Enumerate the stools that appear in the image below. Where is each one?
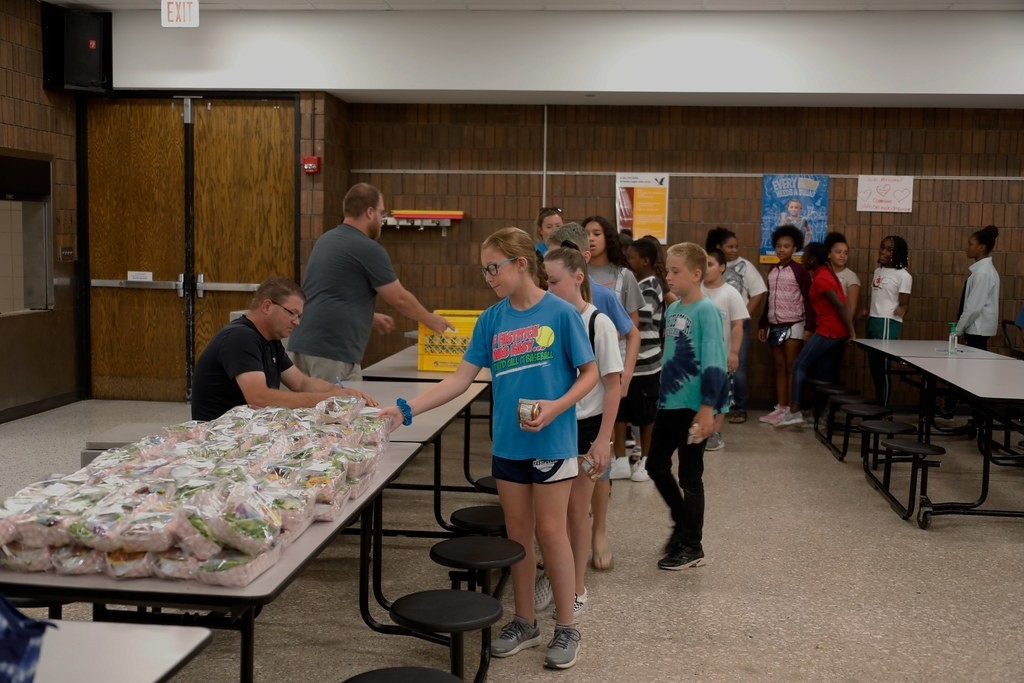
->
[807,378,946,520]
[475,476,498,495]
[429,536,526,595]
[451,506,508,539]
[342,667,464,683]
[389,589,503,679]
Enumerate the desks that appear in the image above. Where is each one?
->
[851,340,1024,530]
[1,342,492,683]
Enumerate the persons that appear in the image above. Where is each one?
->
[780,199,813,248]
[286,183,455,384]
[699,225,861,450]
[533,207,728,622]
[376,228,599,670]
[855,236,912,408]
[192,277,378,422]
[935,224,999,422]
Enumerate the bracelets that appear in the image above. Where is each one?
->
[397,398,412,426]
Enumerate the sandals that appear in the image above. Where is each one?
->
[729,411,747,424]
[714,412,731,419]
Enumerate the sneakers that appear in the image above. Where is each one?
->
[663,522,680,555]
[630,456,650,481]
[553,586,587,619]
[705,432,724,450]
[533,576,553,610]
[609,456,631,479]
[760,404,791,424]
[934,413,955,423]
[773,411,804,429]
[800,416,826,429]
[658,544,706,570]
[490,618,542,657]
[544,623,581,668]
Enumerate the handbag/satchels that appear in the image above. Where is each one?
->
[767,327,787,347]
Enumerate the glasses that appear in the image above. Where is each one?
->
[480,256,518,277]
[271,300,303,322]
[540,207,562,216]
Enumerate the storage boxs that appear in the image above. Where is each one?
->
[418,309,484,371]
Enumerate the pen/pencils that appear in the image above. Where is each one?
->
[336,377,344,389]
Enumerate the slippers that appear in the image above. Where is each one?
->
[592,545,614,573]
[537,559,544,569]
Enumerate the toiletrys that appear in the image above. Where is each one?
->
[947,322,958,354]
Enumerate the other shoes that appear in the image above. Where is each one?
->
[629,446,642,465]
[625,425,636,448]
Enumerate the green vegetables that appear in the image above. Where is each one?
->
[0,401,388,572]
